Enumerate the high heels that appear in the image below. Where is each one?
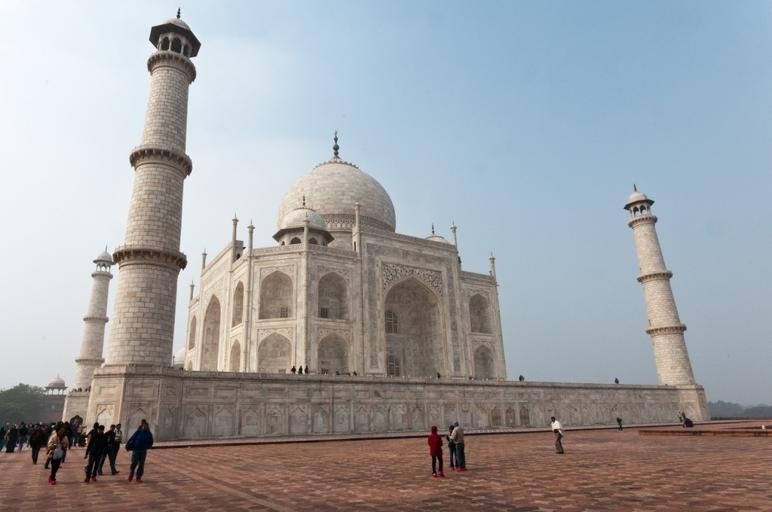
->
[49,475,56,485]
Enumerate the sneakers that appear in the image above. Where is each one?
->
[128,476,133,481]
[438,470,444,477]
[85,477,90,481]
[136,478,144,482]
[92,476,97,481]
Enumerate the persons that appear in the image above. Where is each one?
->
[299,366,302,374]
[519,376,524,381]
[126,420,153,483]
[428,426,444,477]
[449,422,465,472]
[679,412,693,427]
[305,366,308,374]
[447,425,457,468]
[291,366,296,373]
[550,417,564,454]
[617,418,623,431]
[615,378,619,384]
[0,421,123,486]
[437,373,440,379]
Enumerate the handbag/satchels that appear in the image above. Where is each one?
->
[126,430,140,450]
[53,444,64,460]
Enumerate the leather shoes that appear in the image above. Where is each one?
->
[99,472,102,475]
[112,471,119,475]
